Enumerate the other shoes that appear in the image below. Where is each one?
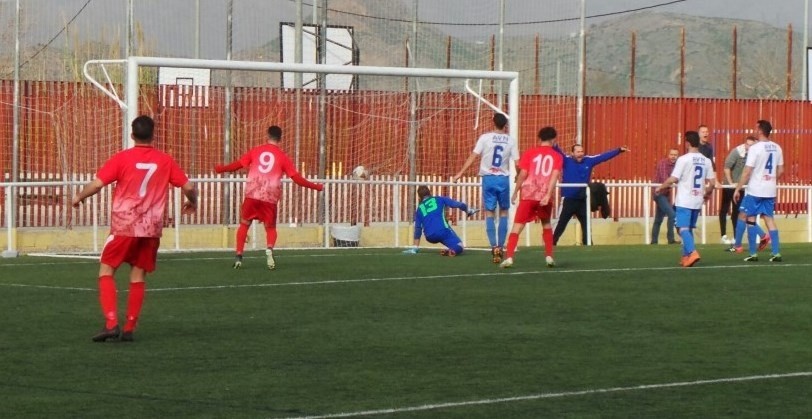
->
[668,240,681,244]
[440,249,456,257]
[492,247,502,263]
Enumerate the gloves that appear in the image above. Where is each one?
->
[403,249,418,255]
[466,208,479,216]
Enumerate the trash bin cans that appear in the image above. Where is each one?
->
[333,224,361,247]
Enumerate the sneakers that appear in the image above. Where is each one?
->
[122,331,132,341]
[545,256,555,267]
[266,247,275,270]
[725,246,742,253]
[757,233,771,251]
[93,323,120,342]
[721,235,736,246]
[745,254,758,262]
[769,254,781,262]
[500,258,513,268]
[680,251,701,267]
[234,256,241,269]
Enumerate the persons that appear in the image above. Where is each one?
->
[655,131,715,268]
[499,126,563,269]
[719,119,785,263]
[697,125,714,163]
[71,115,199,343]
[650,148,682,245]
[553,141,631,246]
[401,187,479,257]
[453,113,520,262]
[213,125,323,270]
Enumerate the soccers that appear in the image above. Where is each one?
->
[353,166,368,180]
